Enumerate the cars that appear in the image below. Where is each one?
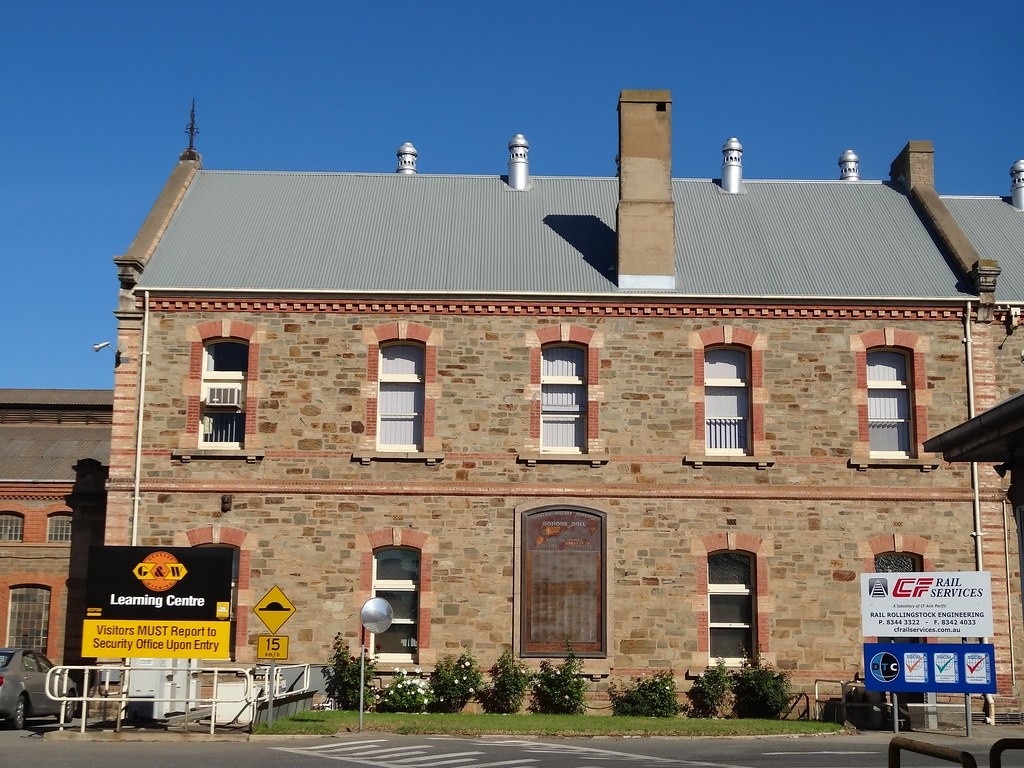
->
[0,648,79,729]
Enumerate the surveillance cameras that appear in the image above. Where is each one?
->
[93,341,110,352]
